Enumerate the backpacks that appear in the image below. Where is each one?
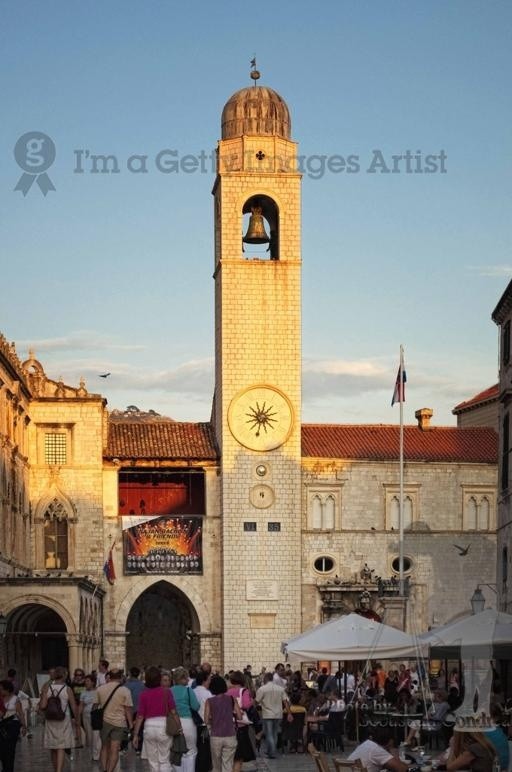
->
[47,686,66,721]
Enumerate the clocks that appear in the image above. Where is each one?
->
[227,383,295,452]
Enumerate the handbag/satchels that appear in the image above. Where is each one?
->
[90,707,104,730]
[166,712,179,737]
[191,709,203,725]
[233,708,254,728]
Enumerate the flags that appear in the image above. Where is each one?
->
[390,355,408,407]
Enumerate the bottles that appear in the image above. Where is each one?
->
[128,550,199,571]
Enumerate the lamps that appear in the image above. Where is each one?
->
[470,583,498,614]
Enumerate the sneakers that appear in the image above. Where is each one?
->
[400,742,411,747]
[410,745,425,752]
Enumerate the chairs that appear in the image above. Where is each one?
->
[252,699,512,772]
